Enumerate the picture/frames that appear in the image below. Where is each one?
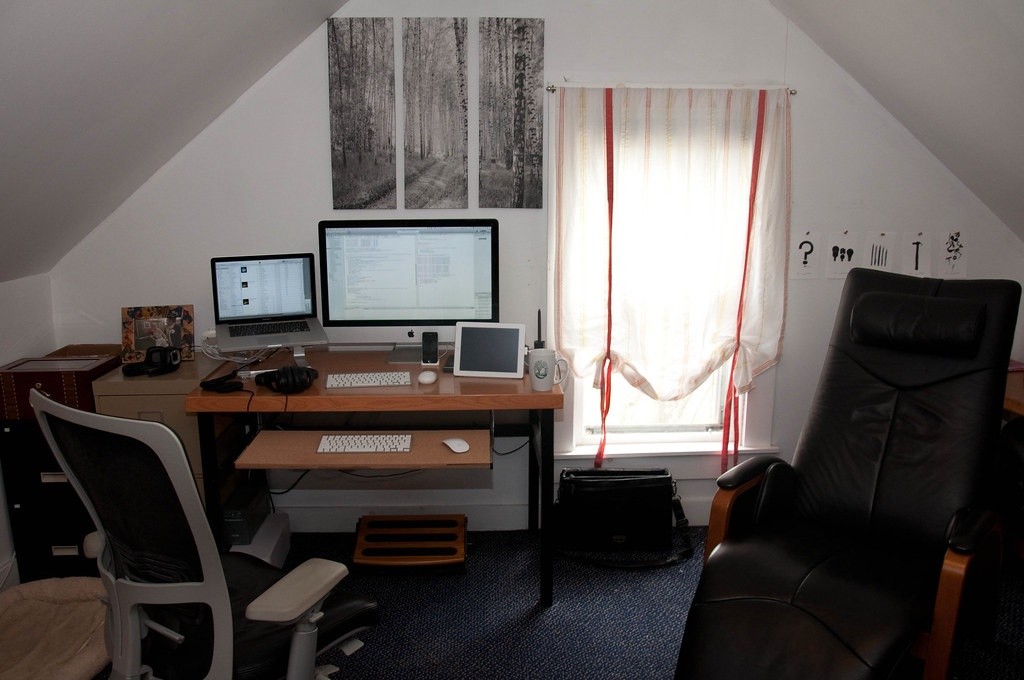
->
[120,305,195,363]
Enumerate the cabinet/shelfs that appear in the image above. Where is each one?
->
[1,415,120,582]
[92,352,234,509]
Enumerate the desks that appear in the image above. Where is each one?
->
[187,345,564,606]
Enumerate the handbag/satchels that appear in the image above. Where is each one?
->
[553,469,707,574]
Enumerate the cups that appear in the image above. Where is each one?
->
[528,349,569,391]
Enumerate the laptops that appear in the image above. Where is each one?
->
[211,252,329,352]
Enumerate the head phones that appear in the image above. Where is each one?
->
[255,366,318,395]
[122,345,181,377]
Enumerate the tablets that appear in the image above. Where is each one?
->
[453,322,526,378]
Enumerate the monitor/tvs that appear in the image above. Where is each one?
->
[318,218,499,363]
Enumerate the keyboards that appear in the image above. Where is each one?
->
[317,435,412,452]
[325,371,411,386]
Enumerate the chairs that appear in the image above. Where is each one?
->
[672,267,1023,680]
[30,389,378,679]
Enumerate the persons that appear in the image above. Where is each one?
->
[150,318,180,348]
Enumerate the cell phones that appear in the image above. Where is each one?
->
[422,332,438,363]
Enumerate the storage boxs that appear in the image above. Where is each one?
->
[0,357,119,420]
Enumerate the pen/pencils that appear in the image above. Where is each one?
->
[236,368,278,376]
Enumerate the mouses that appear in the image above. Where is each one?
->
[442,439,469,453]
[418,371,437,384]
